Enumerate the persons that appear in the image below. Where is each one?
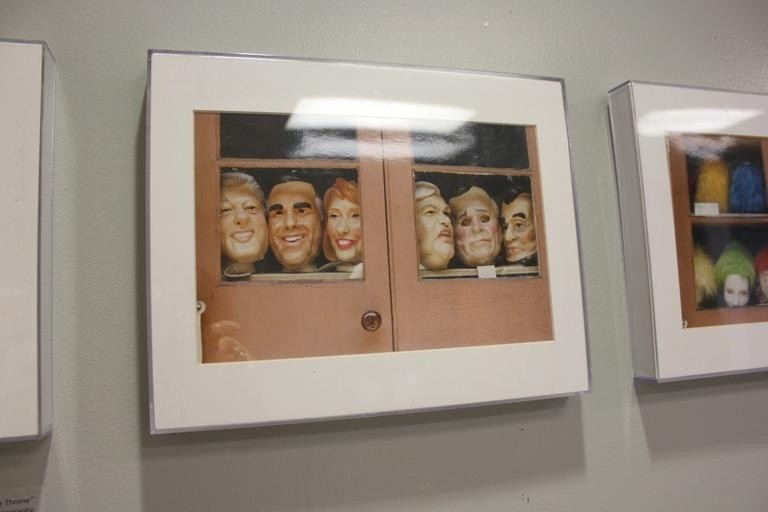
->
[219,170,271,266]
[414,181,455,270]
[448,184,503,268]
[713,239,756,307]
[263,174,323,274]
[208,320,249,362]
[320,177,364,264]
[499,186,538,263]
[752,250,768,304]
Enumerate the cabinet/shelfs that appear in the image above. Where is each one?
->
[192,109,556,367]
[664,130,768,328]
